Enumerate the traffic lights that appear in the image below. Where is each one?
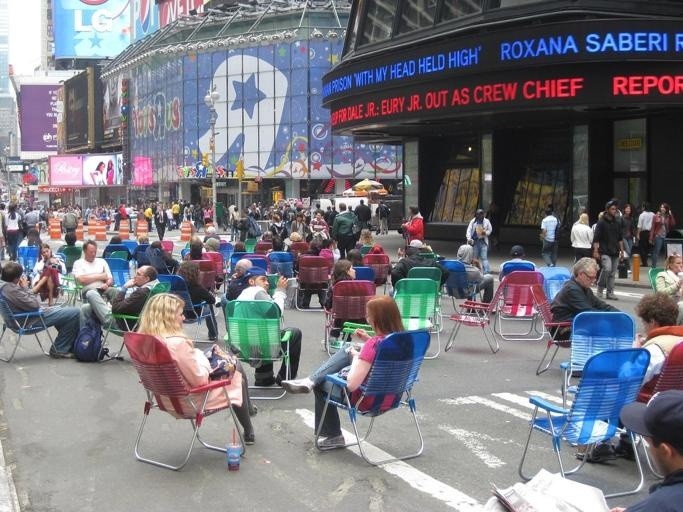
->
[237,160,244,178]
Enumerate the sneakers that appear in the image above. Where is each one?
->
[316,436,346,451]
[606,289,618,300]
[627,270,632,274]
[597,286,605,298]
[50,350,75,358]
[280,377,313,393]
[249,404,258,416]
[244,433,255,444]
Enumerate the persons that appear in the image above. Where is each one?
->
[24,245,68,306]
[15,206,27,214]
[63,208,78,234]
[6,204,22,261]
[324,206,332,219]
[465,209,492,274]
[623,205,635,268]
[232,267,302,386]
[92,162,105,185]
[281,295,414,448]
[71,241,123,332]
[402,204,424,254]
[1,204,5,217]
[324,261,365,340]
[204,227,220,244]
[448,245,496,314]
[357,229,373,247]
[165,200,216,230]
[79,266,158,341]
[241,210,249,241]
[249,198,310,214]
[102,237,132,261]
[205,239,224,288]
[170,261,220,339]
[269,214,286,248]
[540,209,561,268]
[85,204,112,224]
[258,232,273,243]
[74,205,82,219]
[265,238,283,258]
[112,202,154,233]
[37,205,62,224]
[145,246,170,270]
[310,212,328,244]
[332,204,360,259]
[578,292,683,462]
[156,205,167,240]
[134,236,148,258]
[142,241,177,264]
[636,202,655,267]
[230,243,250,255]
[217,203,238,225]
[551,256,624,349]
[376,202,389,234]
[612,390,682,511]
[347,205,355,216]
[354,200,371,230]
[214,259,251,333]
[330,205,339,219]
[230,208,241,241]
[577,206,584,213]
[17,229,43,247]
[393,246,449,297]
[185,238,213,287]
[364,245,392,284]
[499,246,536,314]
[132,292,256,445]
[648,203,676,269]
[657,253,681,321]
[320,239,334,277]
[570,213,593,259]
[589,211,604,235]
[345,249,365,269]
[107,160,114,184]
[315,203,324,217]
[0,262,79,360]
[593,199,626,301]
[291,214,306,241]
[25,209,38,233]
[296,240,329,309]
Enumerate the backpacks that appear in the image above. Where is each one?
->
[246,217,262,237]
[73,312,107,361]
[555,217,564,241]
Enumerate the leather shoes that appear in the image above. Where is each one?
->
[575,443,617,463]
[466,309,478,313]
[547,264,555,267]
[479,309,496,314]
[640,264,649,267]
[612,443,636,461]
[254,376,276,387]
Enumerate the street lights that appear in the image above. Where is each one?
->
[4,146,11,203]
[205,80,220,232]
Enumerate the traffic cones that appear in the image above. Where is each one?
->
[51,222,61,239]
[76,224,84,240]
[95,221,107,241]
[119,220,129,239]
[181,223,192,241]
[88,221,95,235]
[49,219,55,234]
[136,221,148,240]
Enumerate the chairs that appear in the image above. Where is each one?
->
[408,267,444,333]
[0,295,63,363]
[324,280,376,357]
[241,254,267,270]
[15,245,43,279]
[440,260,489,316]
[359,245,372,254]
[269,252,294,286]
[159,240,173,257]
[340,322,377,351]
[107,248,130,263]
[132,244,149,254]
[392,277,440,361]
[499,261,537,277]
[195,249,228,295]
[315,330,430,467]
[125,330,246,472]
[494,270,546,344]
[264,275,283,302]
[184,258,218,318]
[180,248,191,257]
[103,257,131,291]
[254,241,277,253]
[111,242,138,256]
[417,253,437,261]
[348,267,374,282]
[218,241,234,269]
[99,279,171,363]
[559,311,635,414]
[219,237,226,241]
[246,256,268,277]
[230,253,249,277]
[519,348,651,502]
[546,274,572,304]
[445,277,509,354]
[649,266,667,292]
[156,275,218,349]
[295,255,333,312]
[529,283,573,377]
[223,301,292,400]
[639,334,683,402]
[362,253,391,296]
[59,245,81,268]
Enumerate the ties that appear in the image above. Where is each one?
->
[160,211,163,222]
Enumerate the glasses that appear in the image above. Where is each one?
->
[583,272,597,284]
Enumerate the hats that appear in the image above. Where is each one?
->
[408,239,423,249]
[243,267,266,279]
[605,199,617,209]
[620,389,683,445]
[510,245,525,256]
[477,209,485,214]
